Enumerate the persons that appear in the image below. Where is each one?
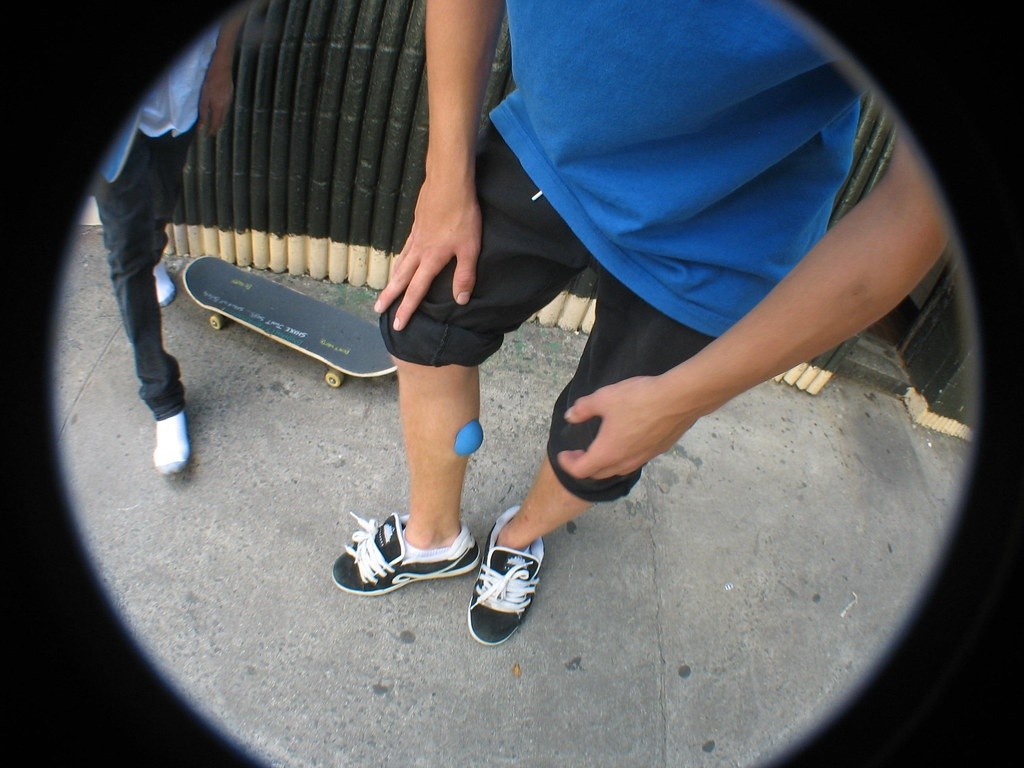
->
[95,5,250,476]
[331,0,949,647]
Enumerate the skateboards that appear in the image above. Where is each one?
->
[183,241,400,389]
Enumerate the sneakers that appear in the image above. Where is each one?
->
[467,505,544,646]
[331,512,480,596]
